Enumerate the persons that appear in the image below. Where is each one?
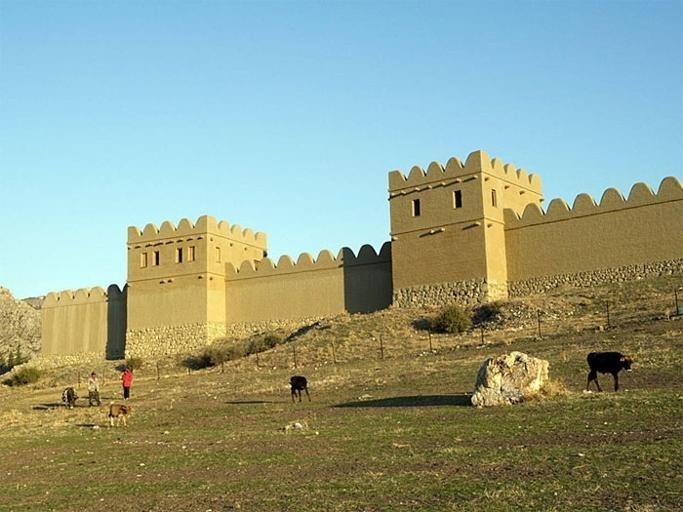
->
[88,371,102,406]
[121,368,133,400]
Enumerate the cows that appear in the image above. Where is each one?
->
[288,375,311,403]
[587,351,636,392]
[108,404,131,427]
